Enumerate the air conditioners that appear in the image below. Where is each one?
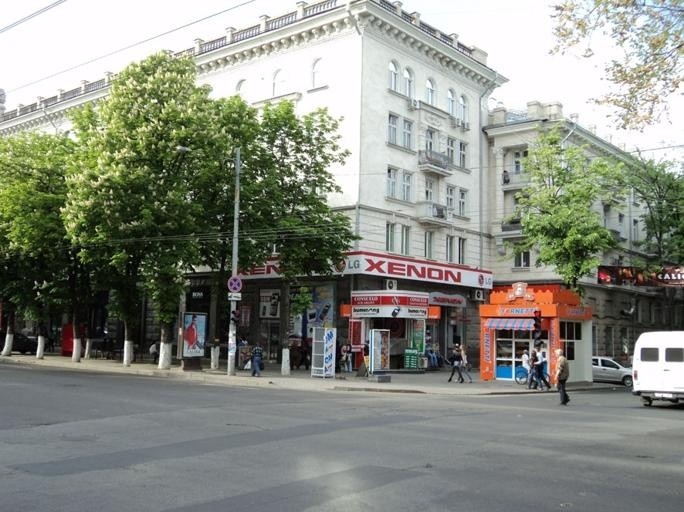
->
[383,278,397,291]
[469,288,483,301]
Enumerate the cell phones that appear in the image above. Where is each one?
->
[270,292,279,316]
[319,303,331,321]
[261,306,267,316]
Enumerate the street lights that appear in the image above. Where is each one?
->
[174,145,242,375]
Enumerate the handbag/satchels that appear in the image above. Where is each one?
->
[260,362,264,370]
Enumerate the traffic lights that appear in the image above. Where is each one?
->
[534,331,543,347]
[229,309,240,324]
[532,310,541,331]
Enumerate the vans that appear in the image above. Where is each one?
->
[630,331,683,407]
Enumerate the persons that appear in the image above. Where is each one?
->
[554,348,570,405]
[521,345,552,392]
[447,341,464,383]
[423,338,444,370]
[184,313,202,351]
[290,340,311,371]
[149,339,158,365]
[455,343,474,383]
[47,329,55,352]
[249,341,262,377]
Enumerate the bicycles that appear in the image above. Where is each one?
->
[514,368,550,387]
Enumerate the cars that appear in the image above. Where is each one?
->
[82,328,123,350]
[592,355,633,387]
[0,328,39,355]
